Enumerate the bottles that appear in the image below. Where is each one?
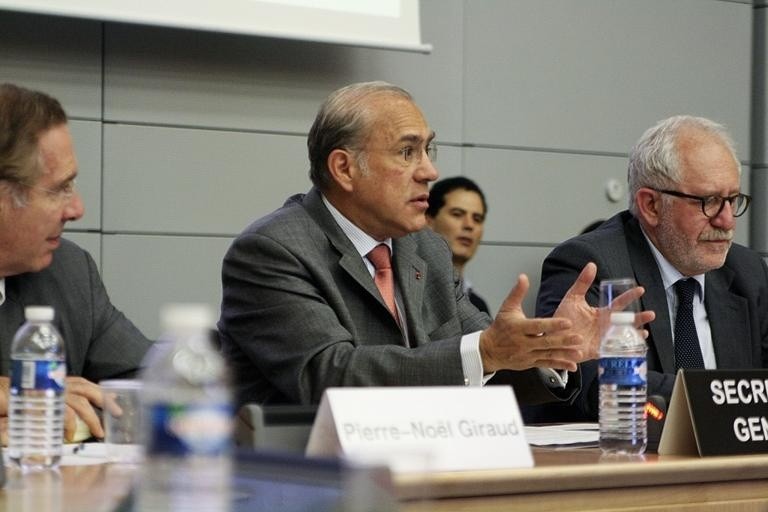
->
[131,301,237,511]
[595,308,649,458]
[5,304,67,475]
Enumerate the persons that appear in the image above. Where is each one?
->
[428,176,491,318]
[541,117,766,451]
[217,84,660,400]
[0,81,160,446]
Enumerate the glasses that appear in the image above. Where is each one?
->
[355,141,440,168]
[649,186,756,222]
[14,177,80,205]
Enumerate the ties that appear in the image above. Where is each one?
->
[670,278,708,376]
[368,244,399,315]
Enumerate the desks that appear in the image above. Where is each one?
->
[0,418,768,511]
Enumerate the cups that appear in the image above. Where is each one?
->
[100,378,145,462]
[597,275,647,347]
